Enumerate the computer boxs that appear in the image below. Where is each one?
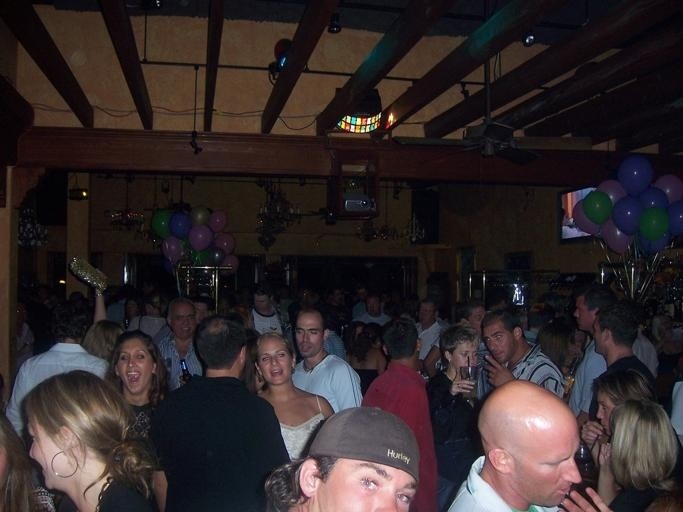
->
[411,190,439,244]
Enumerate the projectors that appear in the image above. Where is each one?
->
[462,123,513,156]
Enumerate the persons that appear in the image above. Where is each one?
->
[0,275,682,512]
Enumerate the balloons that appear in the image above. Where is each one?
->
[568,152,683,265]
[149,202,240,276]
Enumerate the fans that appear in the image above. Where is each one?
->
[392,59,593,167]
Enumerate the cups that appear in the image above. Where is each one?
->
[461,366,475,400]
[563,374,573,394]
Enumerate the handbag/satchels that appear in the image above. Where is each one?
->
[70,256,109,293]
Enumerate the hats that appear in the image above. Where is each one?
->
[309,407,421,483]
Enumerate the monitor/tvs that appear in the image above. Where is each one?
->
[556,187,597,244]
[326,176,376,217]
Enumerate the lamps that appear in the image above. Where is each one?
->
[68,188,87,202]
[520,26,536,48]
[266,13,342,87]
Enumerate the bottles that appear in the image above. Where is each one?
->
[179,360,192,381]
